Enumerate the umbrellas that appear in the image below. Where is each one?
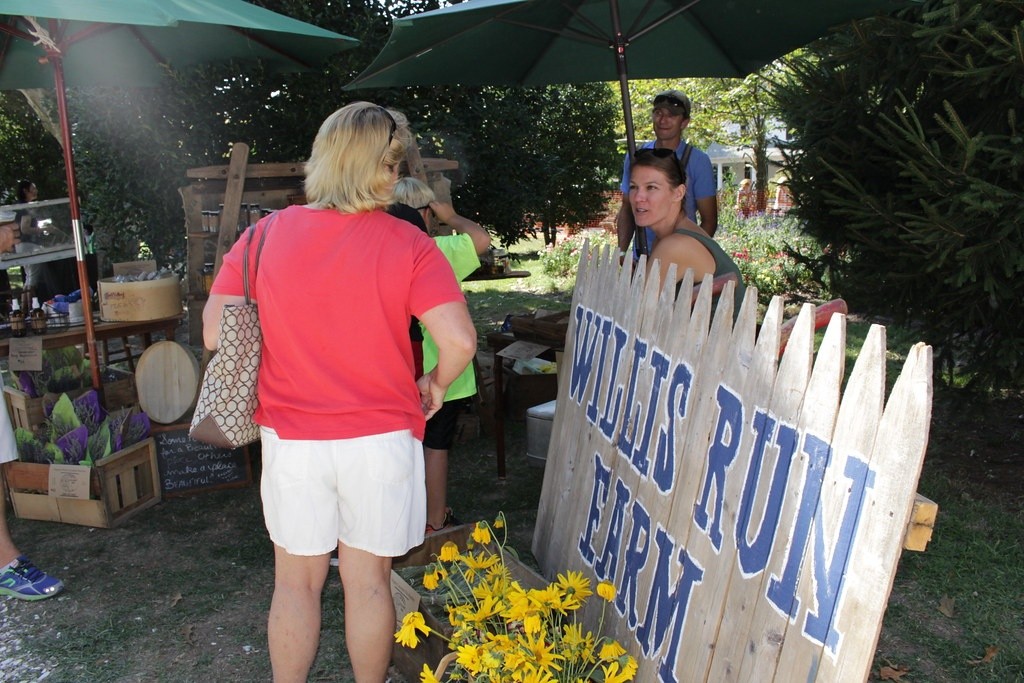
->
[0,0,363,419]
[340,0,909,262]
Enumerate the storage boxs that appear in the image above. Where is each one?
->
[5,435,162,530]
[503,364,558,421]
[391,523,562,676]
[526,398,556,466]
[3,365,135,431]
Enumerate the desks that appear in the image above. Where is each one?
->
[487,330,565,481]
[0,311,187,359]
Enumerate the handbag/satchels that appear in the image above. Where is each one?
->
[188,209,277,449]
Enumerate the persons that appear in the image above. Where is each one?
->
[737,179,752,218]
[628,149,746,333]
[202,102,477,683]
[83,225,99,293]
[617,91,718,283]
[15,180,50,316]
[329,203,428,567]
[774,178,793,216]
[394,177,491,539]
[0,223,65,601]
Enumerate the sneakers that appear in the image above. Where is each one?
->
[0,555,64,601]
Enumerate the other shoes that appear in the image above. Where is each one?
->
[527,400,556,419]
[330,549,338,565]
[426,506,469,535]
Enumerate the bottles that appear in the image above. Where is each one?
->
[11,297,47,337]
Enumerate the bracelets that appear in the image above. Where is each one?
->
[620,252,625,256]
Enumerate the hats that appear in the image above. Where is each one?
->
[652,90,690,116]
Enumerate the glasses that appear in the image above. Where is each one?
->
[416,206,435,218]
[379,105,396,146]
[653,95,687,113]
[633,148,685,182]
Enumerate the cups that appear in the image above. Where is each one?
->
[0,211,17,252]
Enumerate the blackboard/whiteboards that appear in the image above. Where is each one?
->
[150,423,254,499]
